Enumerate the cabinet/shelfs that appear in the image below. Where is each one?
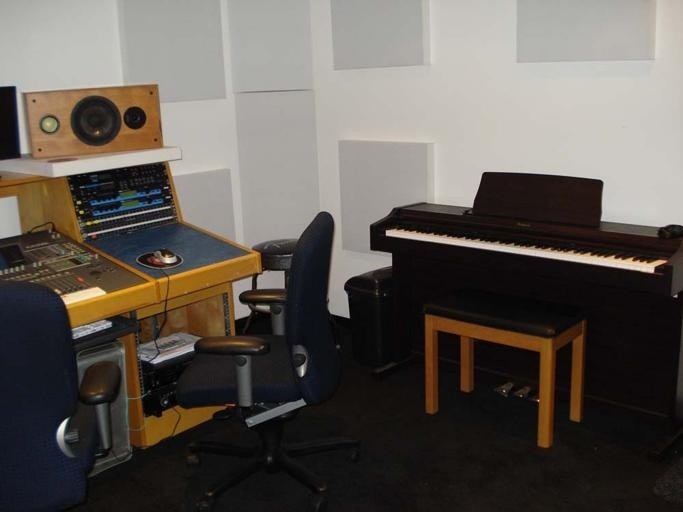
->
[0,149,266,476]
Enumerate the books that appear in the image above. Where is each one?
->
[136,330,203,365]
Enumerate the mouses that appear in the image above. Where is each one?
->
[154,249,177,265]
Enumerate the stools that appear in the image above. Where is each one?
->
[424,283,589,449]
[242,238,296,335]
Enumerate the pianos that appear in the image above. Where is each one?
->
[370,203,683,461]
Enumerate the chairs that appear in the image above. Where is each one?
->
[167,208,341,509]
[1,280,124,510]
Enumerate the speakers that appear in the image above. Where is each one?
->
[20,84,163,159]
